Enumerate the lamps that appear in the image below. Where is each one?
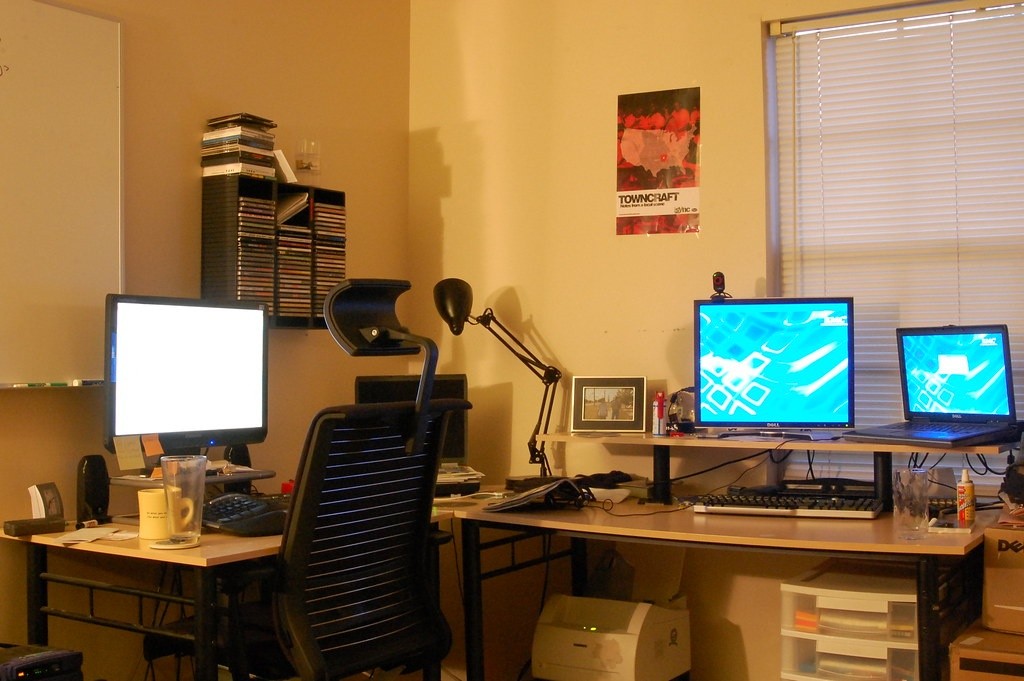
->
[432,278,570,490]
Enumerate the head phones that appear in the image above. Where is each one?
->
[544,494,583,511]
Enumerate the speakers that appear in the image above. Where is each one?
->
[223,443,251,495]
[77,454,109,526]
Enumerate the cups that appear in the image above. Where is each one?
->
[138,488,195,540]
[892,468,929,541]
[160,456,205,543]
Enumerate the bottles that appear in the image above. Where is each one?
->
[652,392,667,436]
[955,470,976,522]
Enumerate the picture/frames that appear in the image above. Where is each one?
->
[570,375,647,433]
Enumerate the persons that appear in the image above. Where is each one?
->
[596,396,621,420]
[618,98,699,186]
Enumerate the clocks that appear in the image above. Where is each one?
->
[28,482,65,519]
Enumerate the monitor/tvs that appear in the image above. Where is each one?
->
[103,293,268,477]
[693,297,855,441]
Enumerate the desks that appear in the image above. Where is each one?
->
[0,482,507,681]
[454,429,1024,681]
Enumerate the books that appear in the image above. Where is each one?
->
[482,478,583,512]
[615,479,684,501]
[436,465,486,483]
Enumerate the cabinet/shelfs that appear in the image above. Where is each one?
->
[780,558,974,680]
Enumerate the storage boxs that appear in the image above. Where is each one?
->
[948,522,1024,680]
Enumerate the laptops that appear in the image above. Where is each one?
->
[354,373,480,484]
[842,323,1024,449]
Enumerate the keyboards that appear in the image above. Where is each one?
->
[202,492,293,536]
[693,494,885,519]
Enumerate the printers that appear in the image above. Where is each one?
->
[530,592,692,681]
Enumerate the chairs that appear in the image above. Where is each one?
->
[141,278,454,681]
